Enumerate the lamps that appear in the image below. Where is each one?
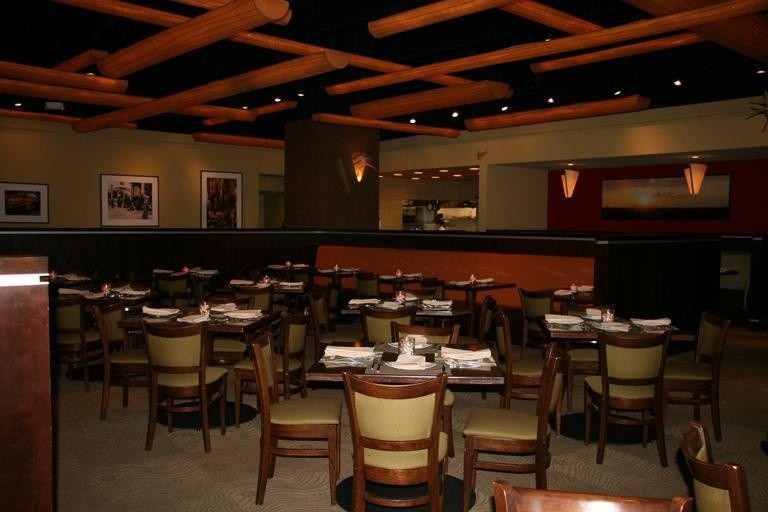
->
[682,161,707,196]
[561,170,580,198]
[351,152,371,183]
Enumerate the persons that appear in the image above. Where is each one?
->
[142,191,150,208]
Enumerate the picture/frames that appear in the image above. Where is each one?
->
[200,170,243,229]
[100,173,159,226]
[0,181,49,223]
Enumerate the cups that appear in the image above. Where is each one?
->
[397,336,416,357]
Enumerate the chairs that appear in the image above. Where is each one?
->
[491,476,694,510]
[52,259,739,467]
[340,371,445,510]
[249,331,343,507]
[681,420,747,510]
[460,340,561,509]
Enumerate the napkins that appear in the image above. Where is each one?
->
[439,344,491,360]
[324,345,375,358]
[394,354,427,371]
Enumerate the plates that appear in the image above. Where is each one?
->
[230,274,304,287]
[350,291,454,309]
[378,269,423,280]
[318,264,358,274]
[146,301,264,324]
[153,265,219,275]
[59,285,148,298]
[386,360,437,371]
[388,343,433,349]
[549,308,670,336]
[268,261,310,270]
